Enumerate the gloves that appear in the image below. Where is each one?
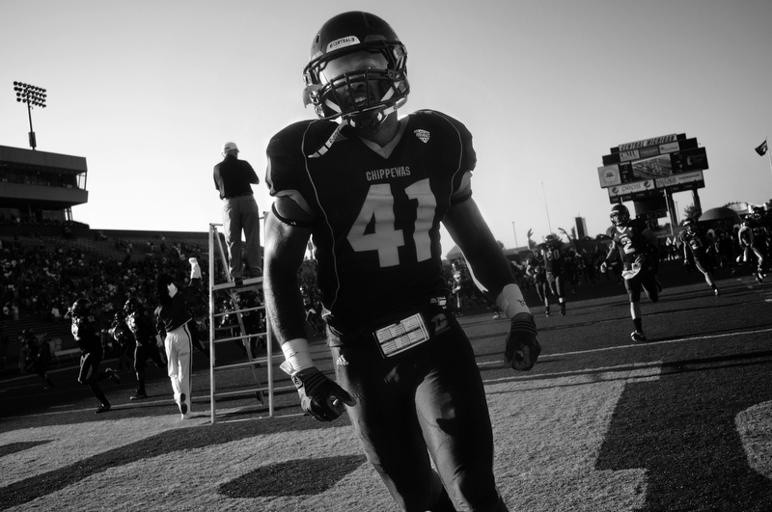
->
[282,352,356,422]
[505,311,543,370]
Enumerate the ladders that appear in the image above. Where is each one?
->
[208,210,298,427]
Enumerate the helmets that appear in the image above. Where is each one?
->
[301,12,409,122]
[609,204,631,227]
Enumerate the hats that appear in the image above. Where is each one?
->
[221,142,241,153]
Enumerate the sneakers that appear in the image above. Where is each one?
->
[174,392,190,417]
[654,281,664,292]
[106,368,121,382]
[130,390,148,400]
[95,402,112,415]
[631,329,647,343]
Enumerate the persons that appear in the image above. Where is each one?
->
[107,296,169,400]
[261,10,541,512]
[214,143,261,284]
[441,201,771,343]
[152,256,206,419]
[0,237,326,394]
[62,296,121,414]
